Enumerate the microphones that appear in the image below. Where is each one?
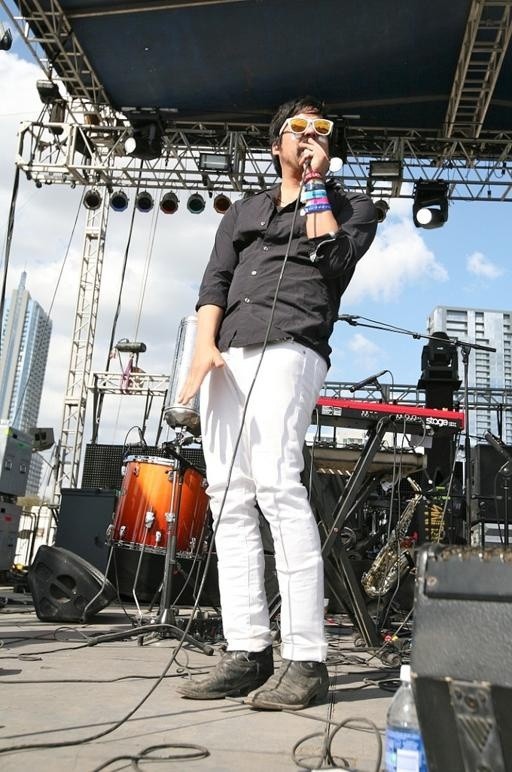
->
[138,429,148,451]
[337,314,360,320]
[350,369,390,393]
[297,136,312,167]
[484,433,512,464]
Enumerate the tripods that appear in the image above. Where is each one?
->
[86,466,214,657]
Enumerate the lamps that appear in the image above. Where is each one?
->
[369,161,401,178]
[116,340,146,353]
[109,191,129,212]
[83,191,102,210]
[412,179,450,231]
[161,192,179,214]
[49,106,64,132]
[135,191,154,213]
[328,148,346,172]
[1,28,13,51]
[37,80,62,101]
[74,132,95,157]
[198,153,233,170]
[214,192,232,213]
[126,113,165,161]
[187,195,206,214]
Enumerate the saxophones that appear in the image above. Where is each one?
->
[361,475,424,599]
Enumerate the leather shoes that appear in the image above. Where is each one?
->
[175,645,273,699]
[247,658,329,709]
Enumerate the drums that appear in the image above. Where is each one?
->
[104,455,212,561]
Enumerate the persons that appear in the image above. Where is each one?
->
[176,94,379,711]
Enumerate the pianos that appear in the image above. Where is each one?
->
[309,396,464,474]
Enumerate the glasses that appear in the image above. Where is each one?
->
[280,116,336,136]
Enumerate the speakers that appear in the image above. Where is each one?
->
[409,541,512,772]
[468,443,512,523]
[26,544,119,623]
[54,487,121,581]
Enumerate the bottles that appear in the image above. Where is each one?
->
[384,662,425,772]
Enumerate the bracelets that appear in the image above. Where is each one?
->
[303,204,332,213]
[302,172,323,183]
[305,189,327,200]
[304,184,325,191]
[306,199,328,206]
